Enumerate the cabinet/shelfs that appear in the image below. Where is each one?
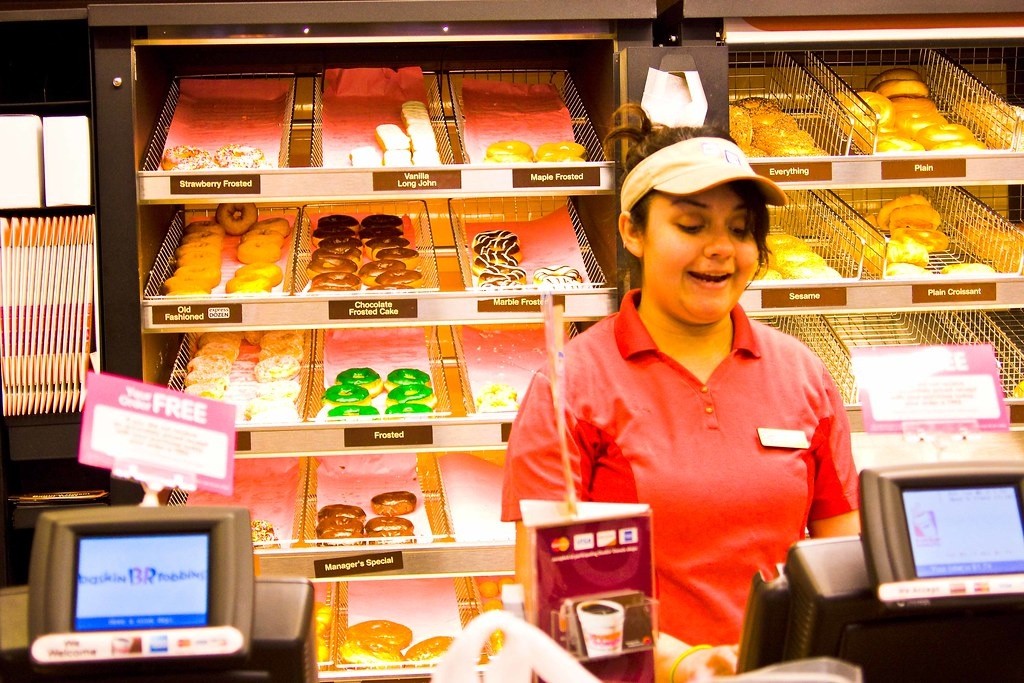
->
[87,0,1024,609]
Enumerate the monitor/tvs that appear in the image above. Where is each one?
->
[26,505,253,673]
[858,461,1024,609]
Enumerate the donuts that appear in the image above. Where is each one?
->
[161,145,273,171]
[476,382,529,411]
[471,229,583,291]
[321,366,438,420]
[339,620,459,670]
[818,193,994,280]
[481,138,588,167]
[723,93,830,161]
[836,67,988,156]
[181,329,307,425]
[306,214,426,291]
[162,202,292,296]
[748,231,846,286]
[251,488,420,545]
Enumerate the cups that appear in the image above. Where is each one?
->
[576,601,625,657]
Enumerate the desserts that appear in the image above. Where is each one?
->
[349,98,441,167]
[480,575,515,612]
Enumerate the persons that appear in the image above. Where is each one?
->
[500,104,864,683]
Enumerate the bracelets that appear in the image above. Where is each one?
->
[670,644,713,683]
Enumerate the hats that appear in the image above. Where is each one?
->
[619,136,788,213]
